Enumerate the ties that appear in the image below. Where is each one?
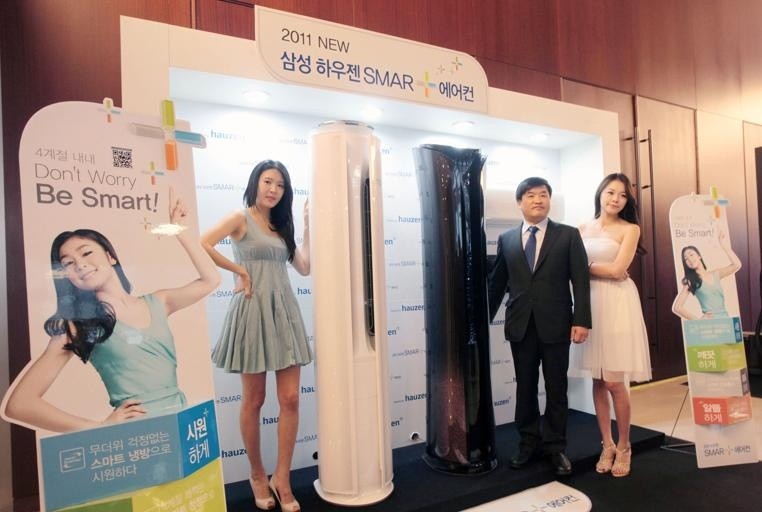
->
[522,226,539,271]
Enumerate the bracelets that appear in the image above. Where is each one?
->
[589,260,593,267]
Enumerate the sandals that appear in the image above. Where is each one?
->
[269,470,302,512]
[249,470,276,511]
[593,439,617,476]
[610,441,632,478]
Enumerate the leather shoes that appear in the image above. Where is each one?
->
[510,452,532,469]
[551,451,572,475]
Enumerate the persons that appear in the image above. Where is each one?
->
[486,177,593,473]
[4,186,221,436]
[670,230,742,321]
[200,157,310,512]
[575,172,651,479]
